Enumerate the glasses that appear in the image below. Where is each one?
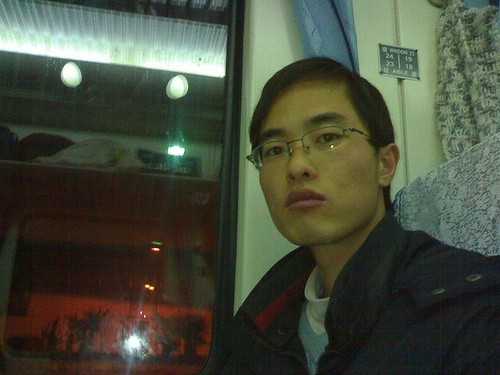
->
[246,125,382,170]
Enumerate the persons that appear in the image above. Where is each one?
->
[203,56,500,375]
